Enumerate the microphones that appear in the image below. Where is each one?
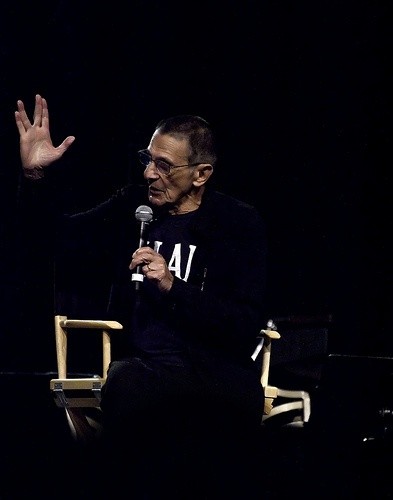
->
[130,205,155,294]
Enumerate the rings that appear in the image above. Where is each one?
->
[147,265,152,272]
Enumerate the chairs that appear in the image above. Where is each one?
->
[257,329,314,431]
[47,314,126,449]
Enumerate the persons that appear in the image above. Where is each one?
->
[13,90,265,500]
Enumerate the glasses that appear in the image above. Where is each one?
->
[138,149,210,176]
